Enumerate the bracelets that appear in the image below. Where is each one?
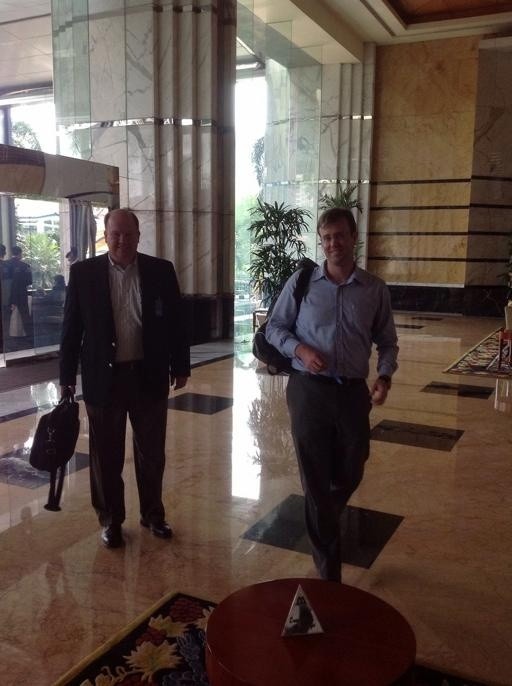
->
[379,376,392,389]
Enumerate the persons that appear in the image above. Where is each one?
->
[1,243,15,350]
[59,208,190,547]
[6,245,33,343]
[264,207,399,583]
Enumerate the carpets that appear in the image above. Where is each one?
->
[51,590,487,686]
[442,327,512,377]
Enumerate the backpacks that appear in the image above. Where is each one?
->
[251,255,321,379]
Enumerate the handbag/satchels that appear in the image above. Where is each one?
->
[26,387,83,511]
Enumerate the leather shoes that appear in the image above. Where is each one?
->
[139,509,174,539]
[99,521,124,549]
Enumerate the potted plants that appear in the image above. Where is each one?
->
[247,197,315,328]
[496,261,512,334]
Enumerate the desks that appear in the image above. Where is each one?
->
[498,331,512,369]
[205,578,416,686]
